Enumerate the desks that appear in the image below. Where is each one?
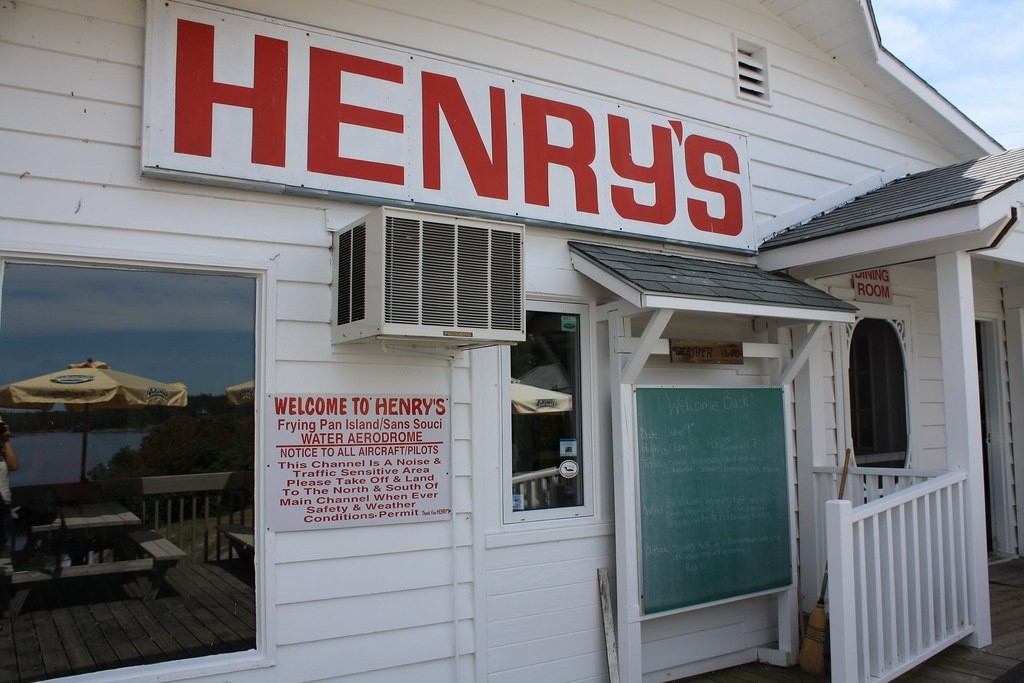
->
[60,501,137,606]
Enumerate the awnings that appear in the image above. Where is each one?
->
[568,242,862,386]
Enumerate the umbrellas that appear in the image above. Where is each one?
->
[226,380,255,404]
[0,358,187,510]
[511,379,572,414]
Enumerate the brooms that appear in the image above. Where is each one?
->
[797,445,852,677]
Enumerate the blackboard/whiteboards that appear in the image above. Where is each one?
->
[632,383,798,620]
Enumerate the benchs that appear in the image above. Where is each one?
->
[0,528,187,585]
[218,523,254,561]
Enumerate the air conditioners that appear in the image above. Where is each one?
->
[332,207,526,351]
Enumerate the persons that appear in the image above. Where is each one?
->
[0,417,20,510]
